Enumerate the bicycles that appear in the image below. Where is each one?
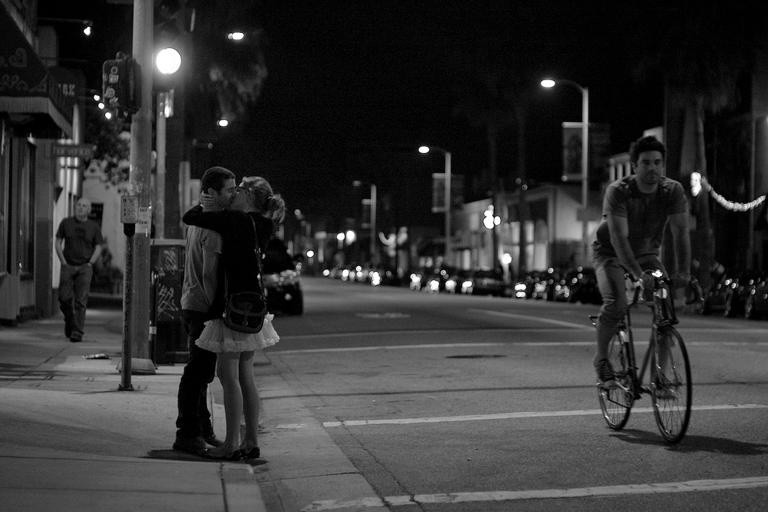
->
[587,267,696,445]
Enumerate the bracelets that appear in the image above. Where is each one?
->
[89,261,93,265]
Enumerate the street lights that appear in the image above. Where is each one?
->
[418,146,451,258]
[539,76,593,264]
[352,181,377,257]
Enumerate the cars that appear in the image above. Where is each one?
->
[331,260,603,305]
[263,242,306,317]
[701,273,768,321]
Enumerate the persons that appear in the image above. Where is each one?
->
[171,166,238,459]
[183,175,286,462]
[592,136,692,400]
[55,197,104,342]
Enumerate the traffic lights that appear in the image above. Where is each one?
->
[153,33,197,91]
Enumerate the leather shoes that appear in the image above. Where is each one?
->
[172,431,261,464]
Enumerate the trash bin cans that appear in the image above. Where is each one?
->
[149,238,189,363]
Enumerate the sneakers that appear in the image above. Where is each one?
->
[70,329,82,342]
[64,320,74,337]
[594,355,617,390]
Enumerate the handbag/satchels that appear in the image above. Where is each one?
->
[221,289,266,335]
